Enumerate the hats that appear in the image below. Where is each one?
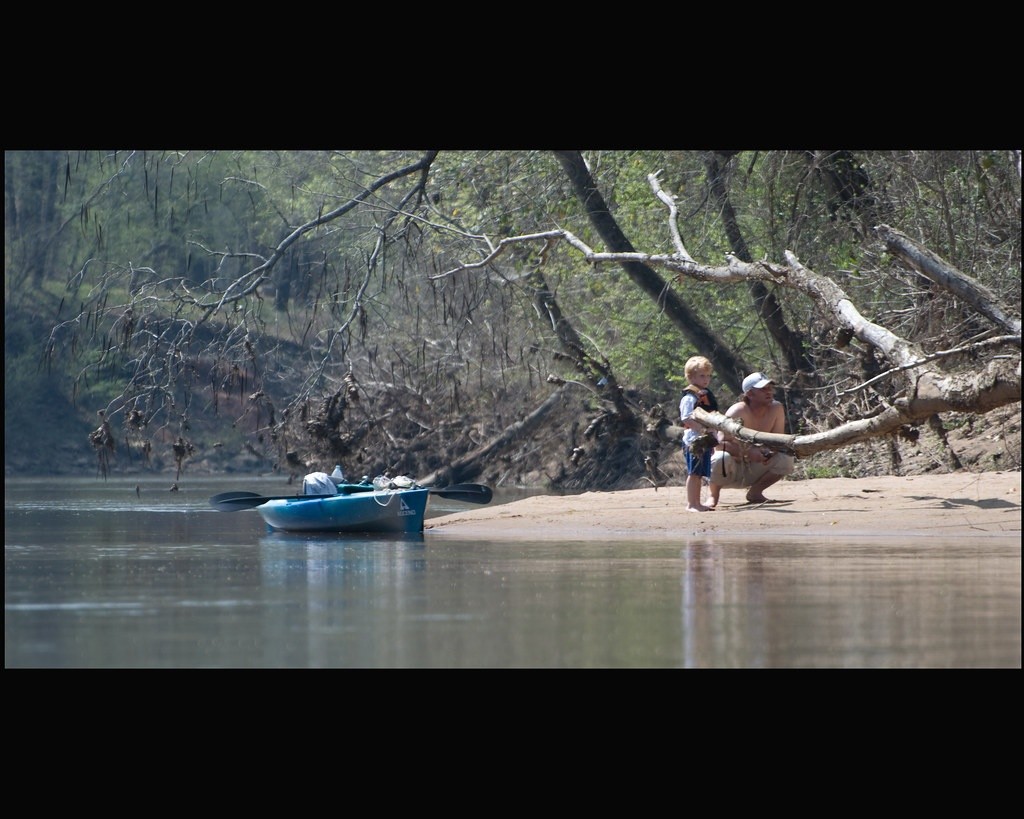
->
[742,371,776,392]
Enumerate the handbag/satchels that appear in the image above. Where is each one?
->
[688,433,720,453]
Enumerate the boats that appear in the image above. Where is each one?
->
[256,475,429,534]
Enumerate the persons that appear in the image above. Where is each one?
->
[701,371,794,508]
[679,355,719,512]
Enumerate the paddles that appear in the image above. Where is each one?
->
[209,484,493,512]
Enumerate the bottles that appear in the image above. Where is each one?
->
[332,464,343,478]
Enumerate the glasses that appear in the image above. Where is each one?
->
[753,385,773,392]
